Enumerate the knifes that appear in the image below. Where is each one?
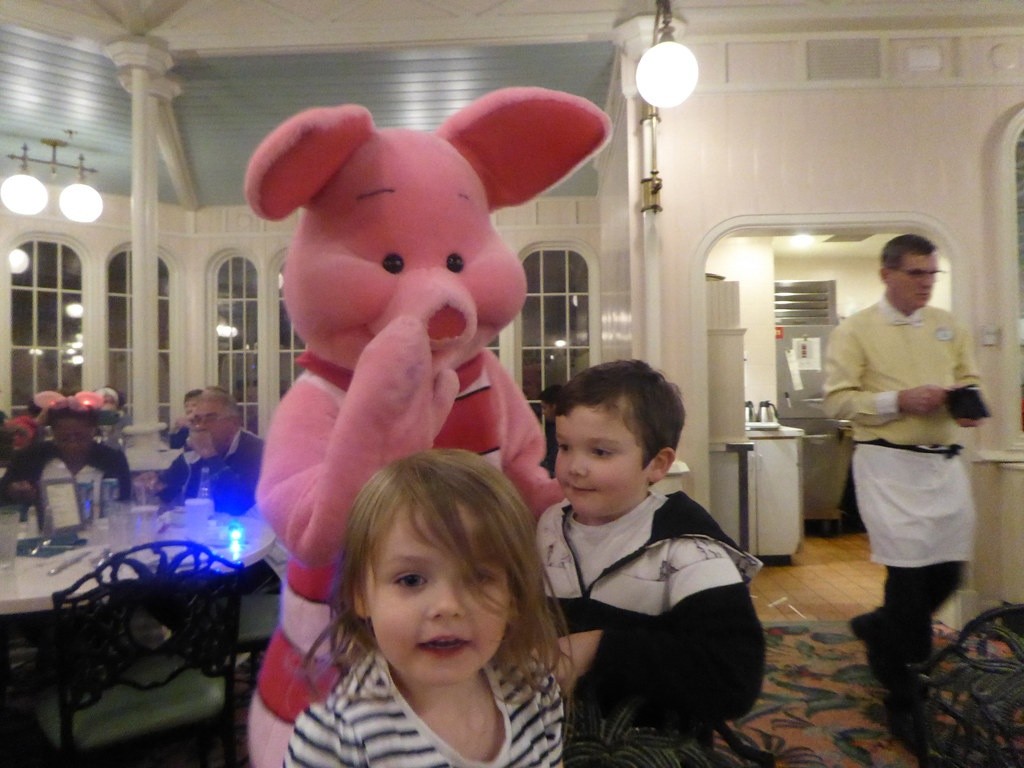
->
[49,551,91,575]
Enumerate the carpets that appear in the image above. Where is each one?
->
[714,612,1024,768]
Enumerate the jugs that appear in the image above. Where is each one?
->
[757,400,777,423]
[745,400,753,422]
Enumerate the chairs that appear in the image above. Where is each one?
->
[25,538,246,768]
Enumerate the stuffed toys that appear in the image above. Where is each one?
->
[242,87,615,768]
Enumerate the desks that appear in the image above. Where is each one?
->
[120,447,185,474]
[0,501,278,616]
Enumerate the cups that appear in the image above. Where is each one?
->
[172,509,184,527]
[110,481,159,548]
[185,499,215,546]
[0,508,21,568]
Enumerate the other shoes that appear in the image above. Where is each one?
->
[850,607,894,688]
[884,676,927,757]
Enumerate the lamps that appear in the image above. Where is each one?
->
[0,136,106,228]
[630,0,701,213]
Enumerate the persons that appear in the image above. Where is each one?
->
[537,384,572,478]
[526,359,767,768]
[280,447,579,768]
[821,231,988,761]
[0,384,265,687]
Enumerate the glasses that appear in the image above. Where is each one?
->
[189,415,225,425]
[896,266,940,278]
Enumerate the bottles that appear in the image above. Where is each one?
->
[27,504,39,538]
[196,466,217,528]
